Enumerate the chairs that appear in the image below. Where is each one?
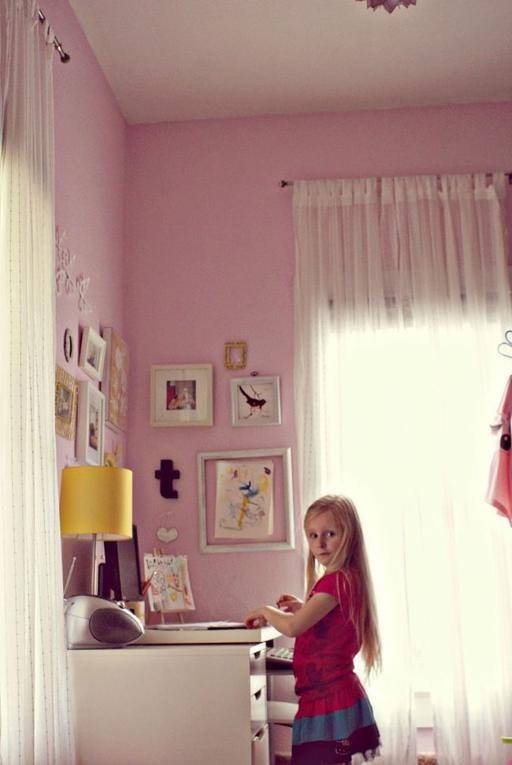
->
[267,699,303,765]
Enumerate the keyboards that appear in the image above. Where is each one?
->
[267,646,294,669]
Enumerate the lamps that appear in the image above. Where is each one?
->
[59,466,133,597]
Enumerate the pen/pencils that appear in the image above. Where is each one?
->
[137,572,155,600]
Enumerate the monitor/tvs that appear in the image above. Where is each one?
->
[104,524,143,602]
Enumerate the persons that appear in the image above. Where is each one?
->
[245,494,384,764]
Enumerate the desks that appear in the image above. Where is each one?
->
[67,622,290,765]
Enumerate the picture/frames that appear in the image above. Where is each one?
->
[194,447,296,555]
[229,375,282,427]
[149,363,214,427]
[56,321,131,467]
